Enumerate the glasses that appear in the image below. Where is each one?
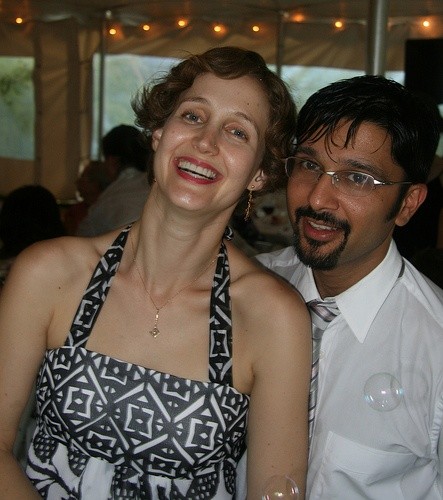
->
[280,156,412,197]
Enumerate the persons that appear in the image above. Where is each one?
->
[1,46,311,500]
[236,74,442,500]
[0,124,443,287]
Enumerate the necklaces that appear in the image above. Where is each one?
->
[128,230,218,338]
